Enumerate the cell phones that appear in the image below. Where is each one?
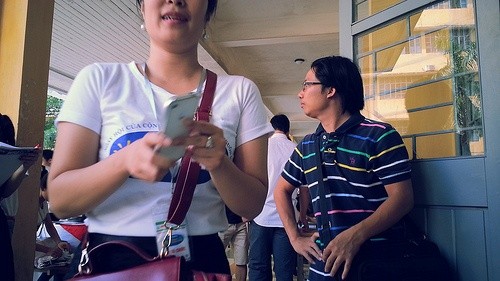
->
[157,93,201,160]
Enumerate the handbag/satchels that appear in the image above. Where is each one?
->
[335,238,451,281]
[68,239,189,281]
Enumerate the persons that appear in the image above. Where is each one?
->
[220,57,416,281]
[48,0,274,281]
[0,113,72,281]
[400,78,456,160]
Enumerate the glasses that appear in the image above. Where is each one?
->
[303,80,323,90]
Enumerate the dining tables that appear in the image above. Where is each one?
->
[51,220,88,249]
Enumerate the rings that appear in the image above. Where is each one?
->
[205,134,214,150]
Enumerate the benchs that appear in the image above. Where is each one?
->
[34,256,73,281]
